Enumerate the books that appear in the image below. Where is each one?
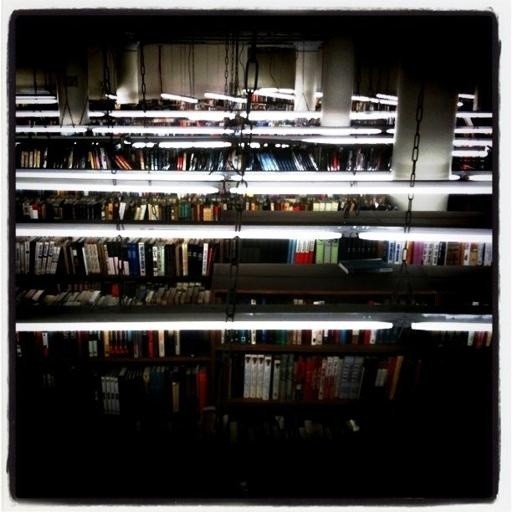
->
[14,96,493,413]
